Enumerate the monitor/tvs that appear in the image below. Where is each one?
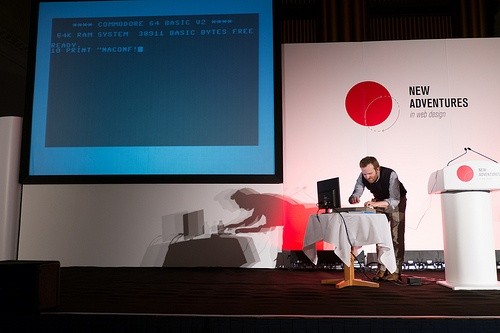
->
[317,177,341,214]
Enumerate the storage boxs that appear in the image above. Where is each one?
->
[0,260,60,312]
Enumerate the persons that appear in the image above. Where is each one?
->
[349,156,407,283]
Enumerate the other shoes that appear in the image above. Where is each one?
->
[372,270,385,281]
[383,273,399,281]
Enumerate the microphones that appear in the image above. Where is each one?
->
[468,147,498,163]
[447,148,467,167]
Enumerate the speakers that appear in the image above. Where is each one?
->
[0,260,60,312]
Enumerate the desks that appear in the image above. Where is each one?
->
[302,213,397,289]
